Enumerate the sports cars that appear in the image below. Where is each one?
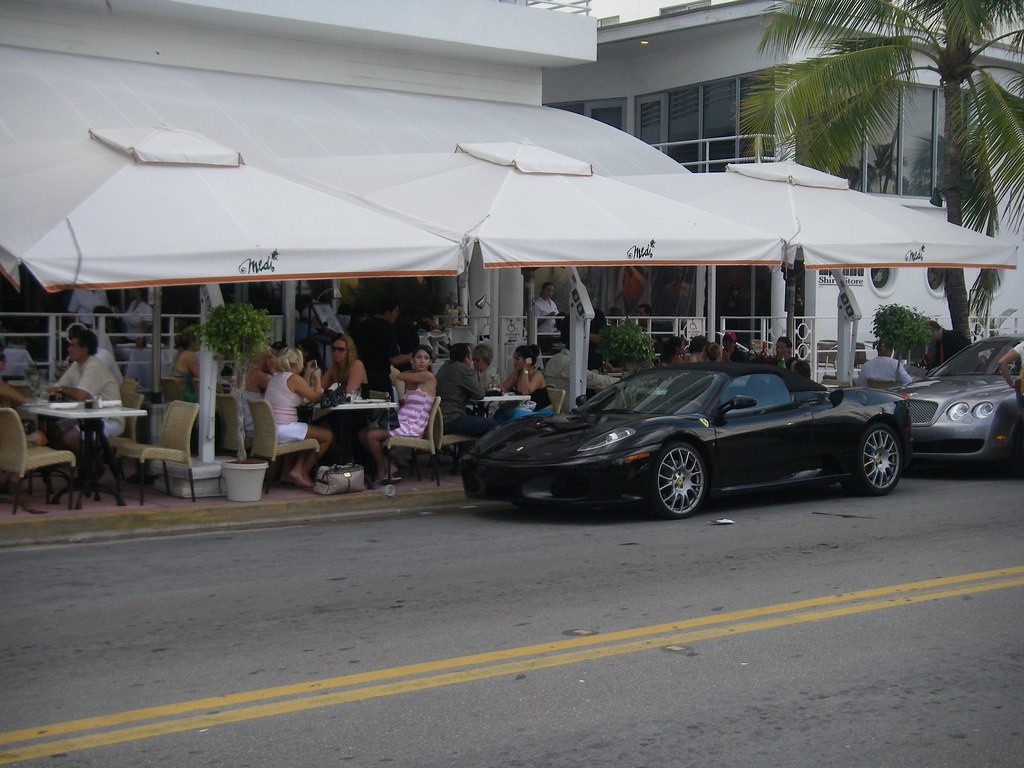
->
[458,361,921,522]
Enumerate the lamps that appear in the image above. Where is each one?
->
[475,295,491,309]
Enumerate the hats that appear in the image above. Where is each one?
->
[723,332,736,341]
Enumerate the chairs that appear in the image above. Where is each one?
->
[349,389,475,487]
[543,383,566,414]
[0,378,321,520]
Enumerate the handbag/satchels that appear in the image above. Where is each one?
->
[500,400,536,411]
[314,463,366,496]
[320,380,346,408]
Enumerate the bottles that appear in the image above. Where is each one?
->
[50,387,64,402]
[327,379,342,393]
[485,375,502,396]
[749,341,775,362]
[85,395,103,409]
[778,356,787,368]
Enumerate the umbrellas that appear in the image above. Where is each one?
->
[0,124,1020,405]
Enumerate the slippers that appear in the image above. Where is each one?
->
[381,475,402,482]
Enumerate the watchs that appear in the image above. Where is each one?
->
[522,370,529,375]
[57,386,63,393]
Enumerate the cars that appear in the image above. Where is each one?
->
[887,333,1024,473]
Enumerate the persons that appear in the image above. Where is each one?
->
[997,340,1024,422]
[923,321,977,376]
[0,281,811,502]
[856,338,914,389]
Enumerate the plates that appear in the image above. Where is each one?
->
[352,400,372,403]
[367,399,385,403]
[49,403,80,409]
[103,400,122,407]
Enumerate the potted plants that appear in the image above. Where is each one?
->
[195,304,272,501]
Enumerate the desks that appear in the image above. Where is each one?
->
[480,393,531,418]
[116,347,181,394]
[305,399,402,487]
[416,322,473,360]
[26,401,147,510]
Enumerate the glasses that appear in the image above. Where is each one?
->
[332,347,344,352]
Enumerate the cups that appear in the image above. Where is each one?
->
[350,388,361,400]
[30,375,41,390]
[379,484,395,497]
[40,392,49,405]
